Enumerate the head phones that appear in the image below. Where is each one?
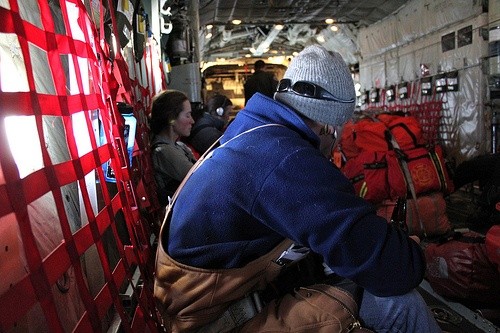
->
[216,96,228,116]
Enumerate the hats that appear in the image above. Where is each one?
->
[273,43,356,125]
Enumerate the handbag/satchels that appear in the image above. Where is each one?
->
[330,114,493,302]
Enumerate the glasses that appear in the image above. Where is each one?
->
[274,79,355,103]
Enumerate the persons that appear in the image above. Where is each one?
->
[149,90,198,205]
[184,94,233,156]
[154,44,440,333]
[243,60,275,107]
